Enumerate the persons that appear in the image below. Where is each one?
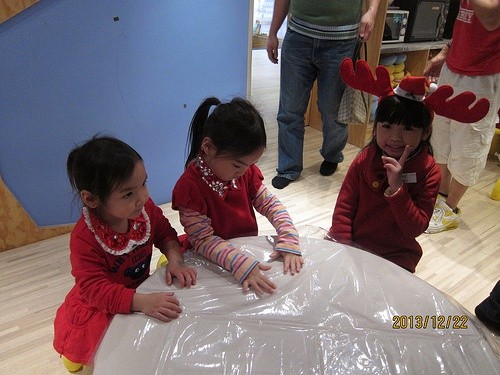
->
[170,96,305,295]
[322,57,489,274]
[266,0,381,189]
[423,0,500,234]
[52,131,198,366]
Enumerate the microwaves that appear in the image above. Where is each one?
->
[405,0,451,42]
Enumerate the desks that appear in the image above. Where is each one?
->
[93,236,500,375]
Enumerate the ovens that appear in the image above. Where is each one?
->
[381,6,410,44]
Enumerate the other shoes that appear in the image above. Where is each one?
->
[474,302,500,328]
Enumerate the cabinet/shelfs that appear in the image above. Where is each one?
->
[305,0,451,150]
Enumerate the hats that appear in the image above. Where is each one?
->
[378,76,434,126]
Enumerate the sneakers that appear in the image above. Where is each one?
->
[424,194,462,233]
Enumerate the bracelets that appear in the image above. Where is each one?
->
[447,41,451,48]
